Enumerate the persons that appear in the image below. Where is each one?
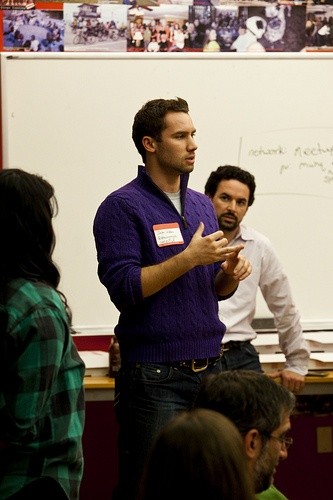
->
[0,0,333,52]
[92,99,252,500]
[0,168,86,500]
[203,166,310,392]
[192,369,294,500]
[140,410,270,500]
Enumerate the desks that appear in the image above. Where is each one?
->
[74,355,332,498]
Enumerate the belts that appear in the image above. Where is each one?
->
[184,354,220,372]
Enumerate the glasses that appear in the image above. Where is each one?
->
[259,432,293,449]
